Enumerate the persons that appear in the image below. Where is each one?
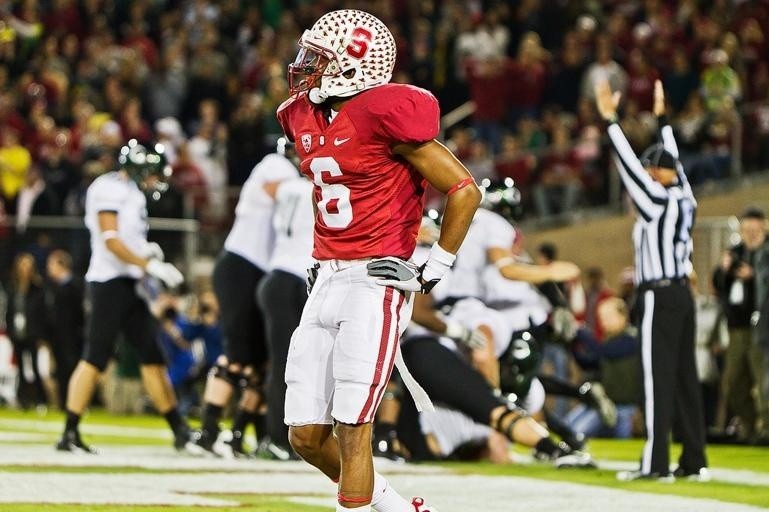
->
[276,8,482,512]
[590,72,717,482]
[2,0,768,471]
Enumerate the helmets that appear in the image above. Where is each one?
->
[116,136,172,204]
[277,134,299,163]
[288,9,397,106]
[481,177,527,221]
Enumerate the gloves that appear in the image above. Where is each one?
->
[367,244,455,296]
[306,264,321,295]
[146,255,185,287]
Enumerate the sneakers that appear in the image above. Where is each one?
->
[673,462,710,482]
[554,448,597,469]
[56,439,101,457]
[173,430,299,461]
[574,378,620,432]
[616,470,673,483]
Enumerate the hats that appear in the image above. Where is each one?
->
[640,144,675,169]
